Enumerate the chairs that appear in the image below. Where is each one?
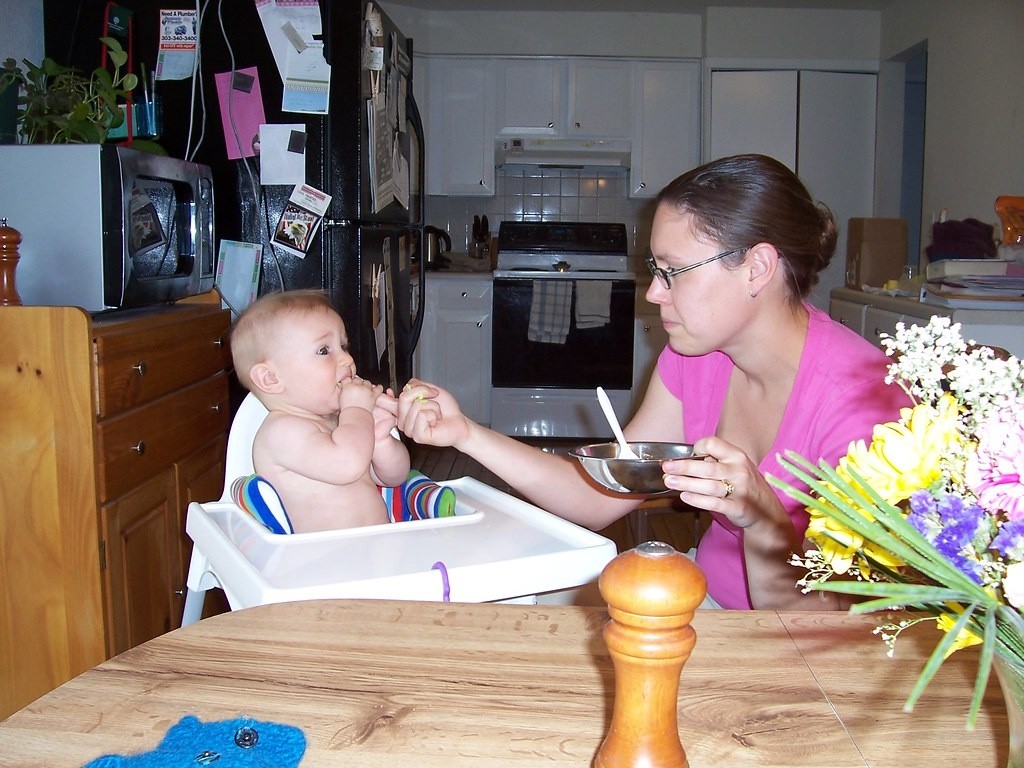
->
[181,389,617,627]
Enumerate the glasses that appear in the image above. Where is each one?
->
[643,246,748,290]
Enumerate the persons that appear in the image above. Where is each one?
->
[231,286,411,534]
[375,154,922,611]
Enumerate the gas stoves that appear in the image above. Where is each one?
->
[497,220,628,273]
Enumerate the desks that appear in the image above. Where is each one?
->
[0,599,1008,768]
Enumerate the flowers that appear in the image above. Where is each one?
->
[763,320,1024,768]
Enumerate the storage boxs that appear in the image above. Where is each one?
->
[845,217,910,289]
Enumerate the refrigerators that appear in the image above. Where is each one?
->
[120,0,430,393]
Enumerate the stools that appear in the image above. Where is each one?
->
[632,497,702,550]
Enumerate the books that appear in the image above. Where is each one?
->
[918,258,1024,310]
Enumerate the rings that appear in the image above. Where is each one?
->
[722,481,734,498]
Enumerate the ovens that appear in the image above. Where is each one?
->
[492,267,636,438]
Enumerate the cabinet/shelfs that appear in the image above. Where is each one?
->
[829,290,1024,367]
[0,290,241,723]
[413,275,670,437]
[410,53,704,201]
[702,56,882,317]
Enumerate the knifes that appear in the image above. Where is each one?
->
[472,212,490,241]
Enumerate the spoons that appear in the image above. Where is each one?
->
[597,387,638,459]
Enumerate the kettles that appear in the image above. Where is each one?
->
[423,224,451,269]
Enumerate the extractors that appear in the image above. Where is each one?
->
[494,134,630,169]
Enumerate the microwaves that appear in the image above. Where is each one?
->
[0,144,215,311]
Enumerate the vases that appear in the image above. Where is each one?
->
[991,651,1024,768]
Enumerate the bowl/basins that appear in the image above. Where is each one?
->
[567,443,710,494]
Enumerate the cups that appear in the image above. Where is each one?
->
[883,280,898,290]
[903,265,914,277]
